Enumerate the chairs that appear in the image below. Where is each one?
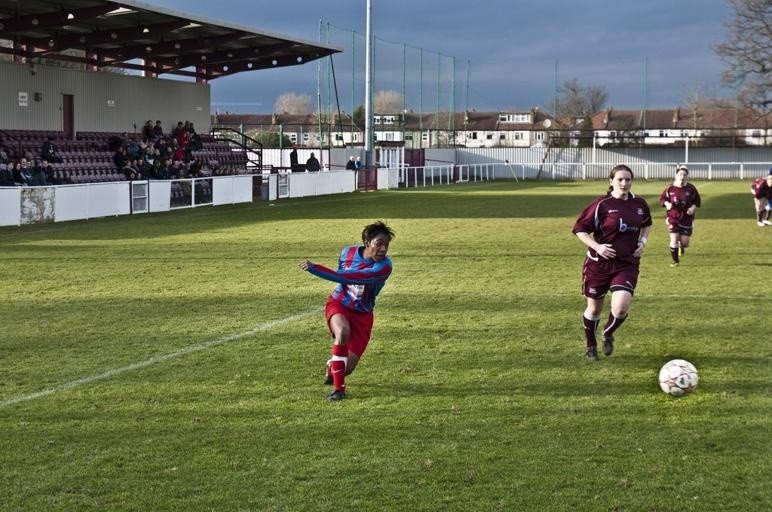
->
[0,128,249,184]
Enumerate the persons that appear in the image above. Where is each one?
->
[289,145,299,167]
[297,221,395,402]
[113,118,242,180]
[765,167,771,221]
[305,152,321,171]
[344,155,356,170]
[354,156,362,171]
[2,135,63,186]
[749,175,771,227]
[571,164,655,364]
[659,166,701,268]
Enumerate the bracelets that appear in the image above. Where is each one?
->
[638,235,647,247]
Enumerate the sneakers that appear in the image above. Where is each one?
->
[670,260,678,267]
[678,247,684,256]
[324,359,332,384]
[328,390,345,400]
[602,330,612,355]
[757,220,772,226]
[586,347,598,361]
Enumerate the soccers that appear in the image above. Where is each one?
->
[659,359,698,396]
